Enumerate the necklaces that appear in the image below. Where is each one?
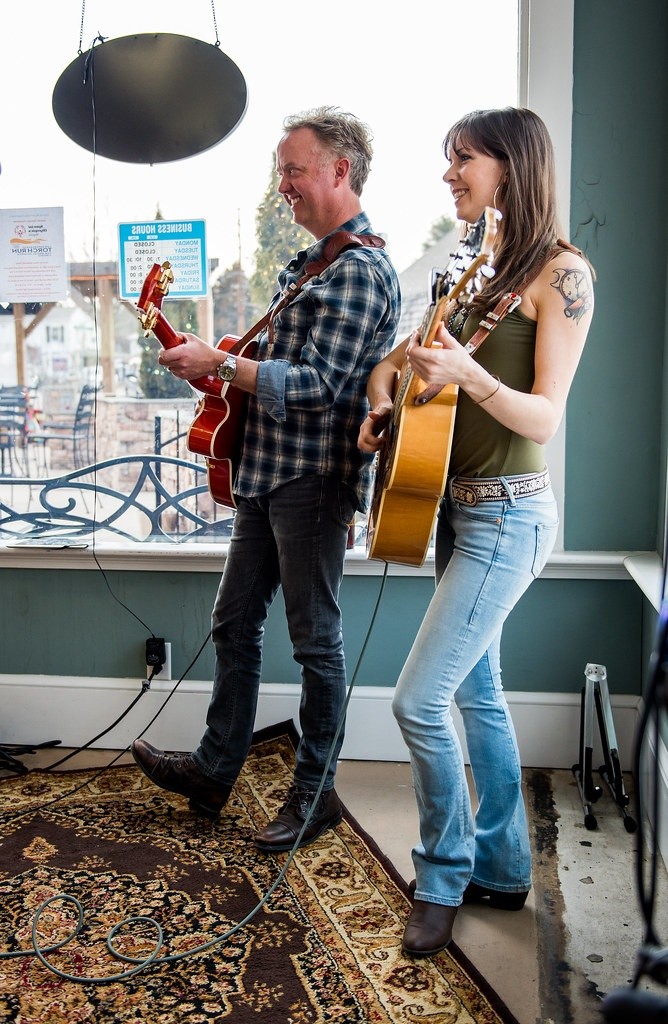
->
[448,303,474,336]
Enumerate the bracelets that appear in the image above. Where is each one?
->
[472,374,501,404]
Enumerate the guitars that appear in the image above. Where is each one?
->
[367,208,504,567]
[135,259,260,508]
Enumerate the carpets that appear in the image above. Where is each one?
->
[0,717,520,1024]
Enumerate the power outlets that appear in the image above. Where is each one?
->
[147,643,172,680]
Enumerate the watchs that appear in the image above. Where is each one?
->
[216,352,237,382]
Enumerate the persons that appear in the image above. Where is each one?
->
[355,107,597,955]
[131,105,401,854]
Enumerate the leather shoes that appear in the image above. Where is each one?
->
[254,786,343,852]
[402,899,459,958]
[131,738,233,821]
[408,878,529,911]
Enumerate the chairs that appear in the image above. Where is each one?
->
[0,384,103,513]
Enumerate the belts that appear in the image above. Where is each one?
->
[448,472,551,507]
